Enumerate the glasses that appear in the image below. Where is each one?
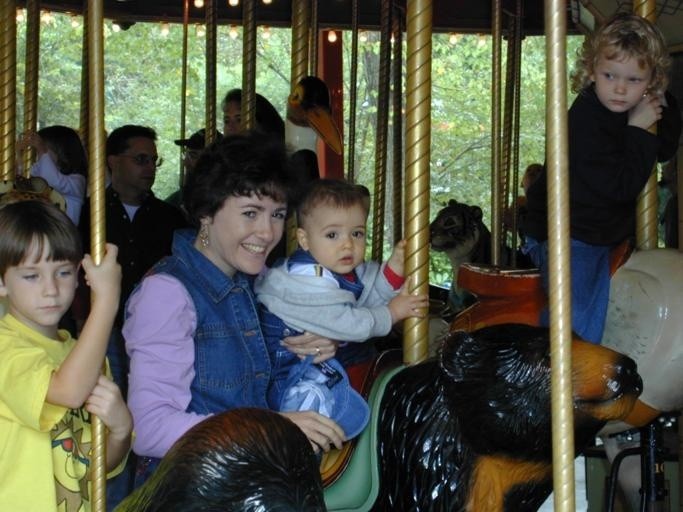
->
[123,152,163,168]
[180,150,200,161]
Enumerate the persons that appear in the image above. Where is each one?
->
[120,131,347,493]
[71,125,187,510]
[505,164,543,251]
[517,14,682,344]
[0,200,135,511]
[175,127,224,175]
[15,126,86,226]
[222,89,285,145]
[658,157,683,248]
[251,177,430,372]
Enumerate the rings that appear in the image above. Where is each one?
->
[315,347,324,357]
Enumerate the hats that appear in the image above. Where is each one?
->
[270,349,374,444]
